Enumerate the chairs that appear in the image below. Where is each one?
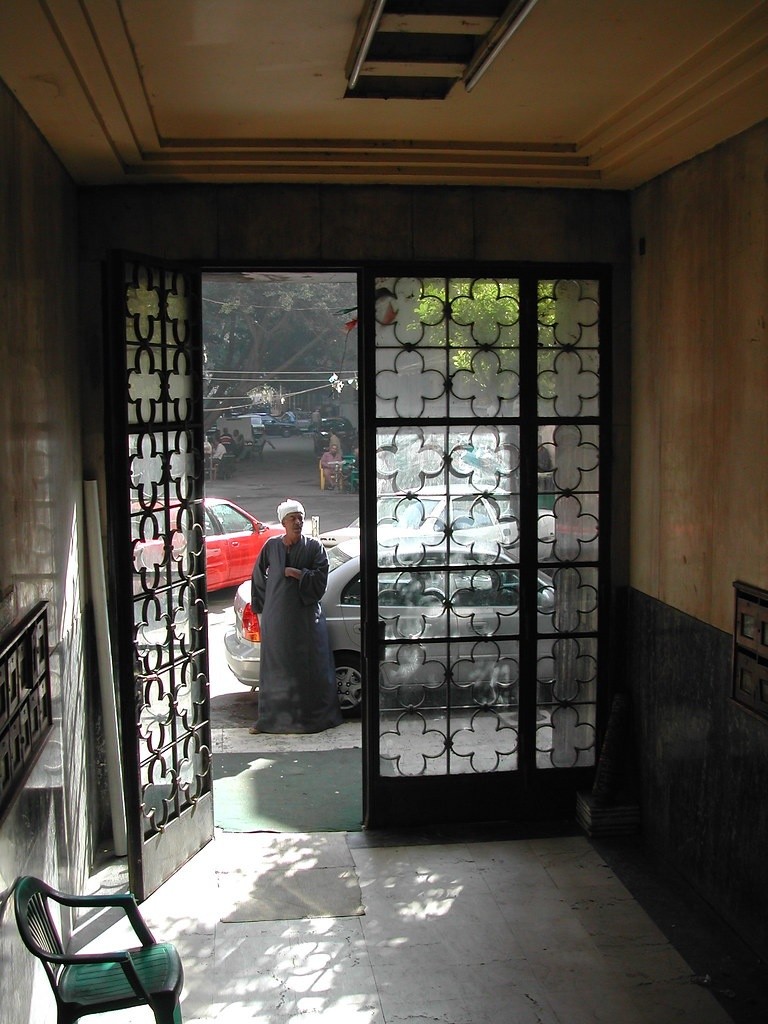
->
[250,439,267,457]
[319,460,343,490]
[212,453,236,481]
[13,875,184,1024]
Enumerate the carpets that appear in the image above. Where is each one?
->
[212,748,398,835]
[219,830,368,923]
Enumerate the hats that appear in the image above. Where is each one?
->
[277,499,305,523]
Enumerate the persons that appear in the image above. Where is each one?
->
[251,499,346,735]
[537,433,553,471]
[325,428,342,458]
[280,408,296,422]
[204,427,244,473]
[321,445,343,490]
[311,410,321,424]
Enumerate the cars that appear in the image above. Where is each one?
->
[313,417,357,455]
[130,497,285,628]
[236,408,317,437]
[224,481,556,710]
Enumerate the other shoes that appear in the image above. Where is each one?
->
[249,726,261,734]
[328,486,334,489]
